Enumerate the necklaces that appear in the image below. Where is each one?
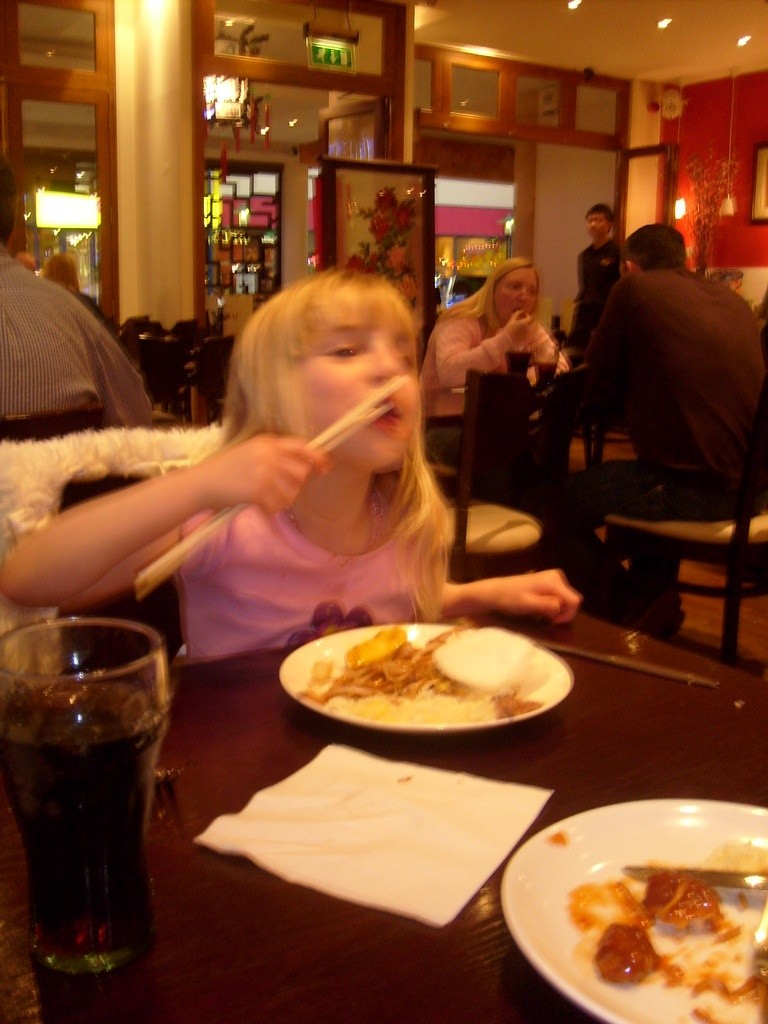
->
[286,489,384,549]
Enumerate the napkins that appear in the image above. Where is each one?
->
[193,744,553,927]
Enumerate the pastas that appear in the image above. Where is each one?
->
[295,625,457,702]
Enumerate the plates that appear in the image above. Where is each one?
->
[280,623,573,731]
[502,797,768,1024]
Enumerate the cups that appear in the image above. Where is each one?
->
[0,620,165,1024]
[504,345,532,378]
[536,346,560,384]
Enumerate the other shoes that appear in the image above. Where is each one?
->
[618,584,681,639]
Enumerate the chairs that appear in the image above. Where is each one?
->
[119,316,236,429]
[451,368,768,664]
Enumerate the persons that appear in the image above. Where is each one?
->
[0,154,768,641]
[0,263,583,669]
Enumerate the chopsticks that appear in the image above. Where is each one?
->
[132,378,408,598]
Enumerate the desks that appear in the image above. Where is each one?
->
[421,385,464,466]
[1,600,768,1024]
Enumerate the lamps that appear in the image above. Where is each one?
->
[204,21,269,137]
[719,65,735,216]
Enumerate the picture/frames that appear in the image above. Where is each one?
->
[320,156,440,372]
[750,141,768,225]
